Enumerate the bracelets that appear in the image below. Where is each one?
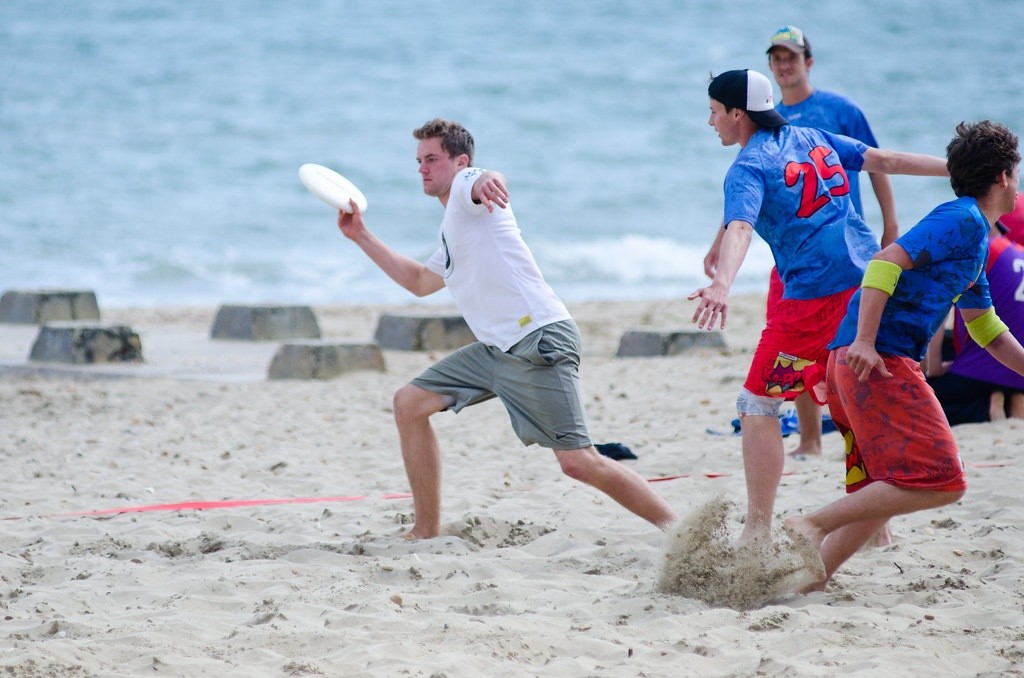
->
[965,307,1007,348]
[861,260,902,296]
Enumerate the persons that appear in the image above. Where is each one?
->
[784,122,1024,594]
[686,26,1024,549]
[339,119,681,542]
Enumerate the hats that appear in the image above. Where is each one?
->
[709,68,788,128]
[765,25,810,54]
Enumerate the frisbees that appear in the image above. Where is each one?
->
[297,162,366,215]
